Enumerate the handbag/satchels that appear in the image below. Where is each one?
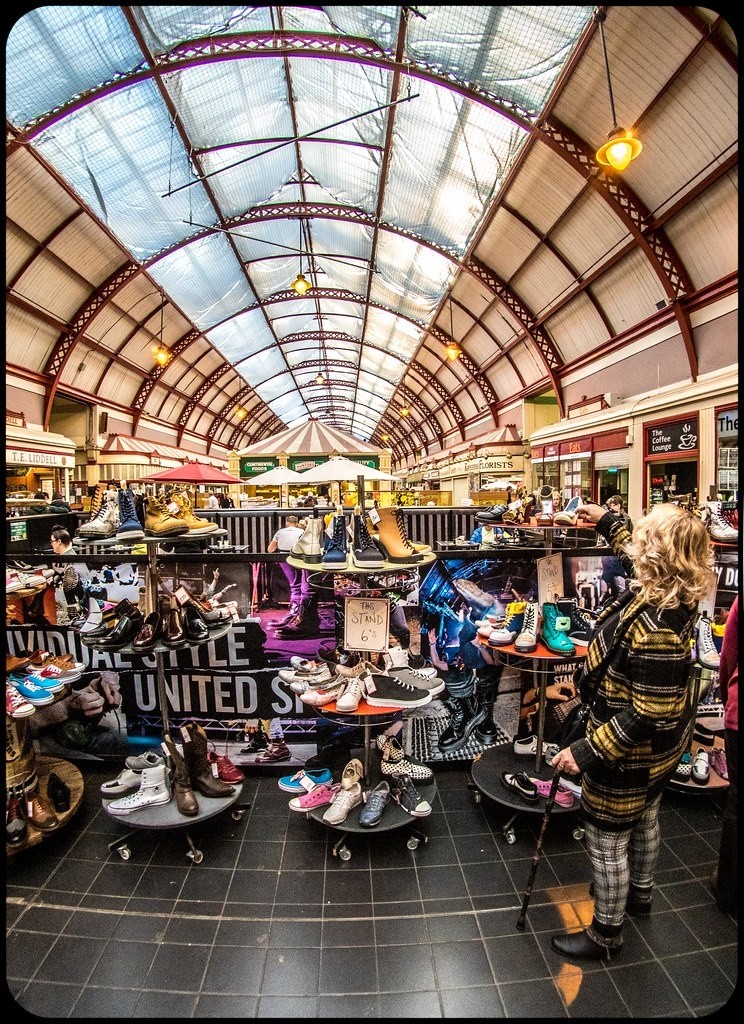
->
[559,703,590,786]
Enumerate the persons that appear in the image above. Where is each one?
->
[26,491,91,583]
[368,571,502,753]
[241,716,359,768]
[548,490,738,959]
[505,486,512,505]
[470,525,503,550]
[201,566,237,607]
[521,682,577,718]
[27,671,121,729]
[269,562,322,640]
[205,489,378,552]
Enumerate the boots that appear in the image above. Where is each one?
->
[702,492,738,543]
[690,610,722,670]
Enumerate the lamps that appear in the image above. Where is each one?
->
[381,425,389,441]
[153,290,172,365]
[592,5,642,171]
[314,314,325,385]
[444,288,463,360]
[234,375,249,420]
[400,374,410,416]
[289,216,312,295]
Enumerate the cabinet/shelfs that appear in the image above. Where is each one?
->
[469,520,586,845]
[285,552,439,860]
[72,529,245,864]
[668,539,738,796]
[6,582,86,857]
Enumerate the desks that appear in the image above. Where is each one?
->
[34,546,92,553]
[209,544,250,553]
[435,541,480,550]
[98,546,131,554]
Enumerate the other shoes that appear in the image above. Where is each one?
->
[673,752,691,782]
[692,746,709,784]
[692,722,715,747]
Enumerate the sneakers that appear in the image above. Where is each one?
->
[6,484,591,837]
[710,749,729,780]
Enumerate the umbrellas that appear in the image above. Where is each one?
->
[481,478,518,490]
[137,458,399,507]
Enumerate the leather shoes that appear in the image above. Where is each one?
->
[588,881,652,918]
[550,922,621,962]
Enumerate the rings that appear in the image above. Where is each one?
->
[558,763,564,768]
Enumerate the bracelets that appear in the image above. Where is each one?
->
[429,642,436,645]
[214,577,218,581]
[535,687,540,697]
